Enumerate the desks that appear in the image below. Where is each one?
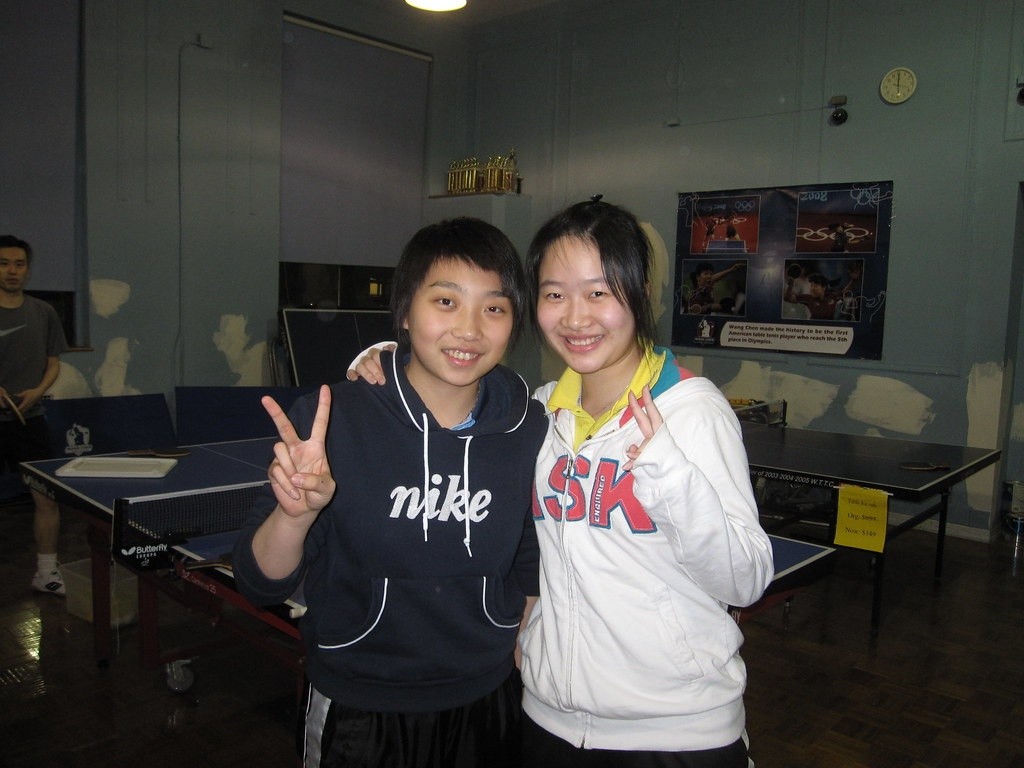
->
[732,420,1004,665]
[18,435,837,738]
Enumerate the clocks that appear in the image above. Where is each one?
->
[880,67,917,104]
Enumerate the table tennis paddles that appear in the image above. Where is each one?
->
[899,460,952,470]
[4,392,28,427]
[182,548,235,572]
[788,263,801,278]
[719,298,732,312]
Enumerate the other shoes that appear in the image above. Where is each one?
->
[33,569,66,596]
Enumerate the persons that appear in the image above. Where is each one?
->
[702,214,718,247]
[230,216,549,768]
[828,221,854,252]
[784,274,836,320]
[683,263,746,314]
[347,201,775,768]
[0,234,71,594]
[720,214,747,240]
